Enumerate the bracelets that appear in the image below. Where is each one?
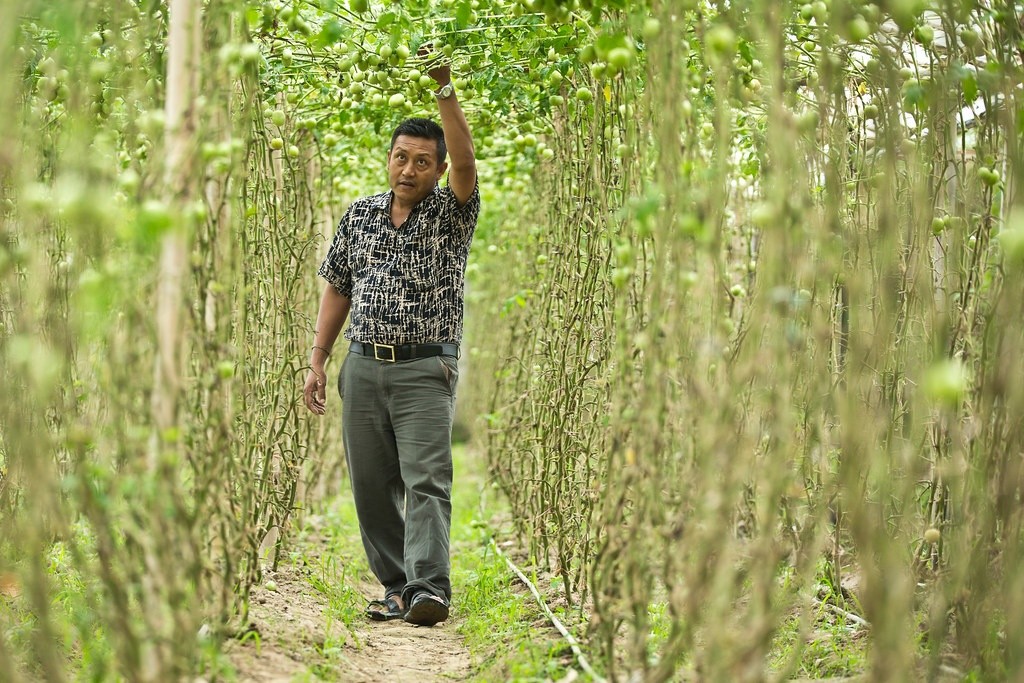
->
[311,345,330,356]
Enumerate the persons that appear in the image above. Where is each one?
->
[299,38,483,627]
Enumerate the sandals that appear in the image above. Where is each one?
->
[403,590,449,627]
[364,593,410,621]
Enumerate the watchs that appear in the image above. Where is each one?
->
[432,80,455,100]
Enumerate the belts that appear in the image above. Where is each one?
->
[348,340,458,363]
